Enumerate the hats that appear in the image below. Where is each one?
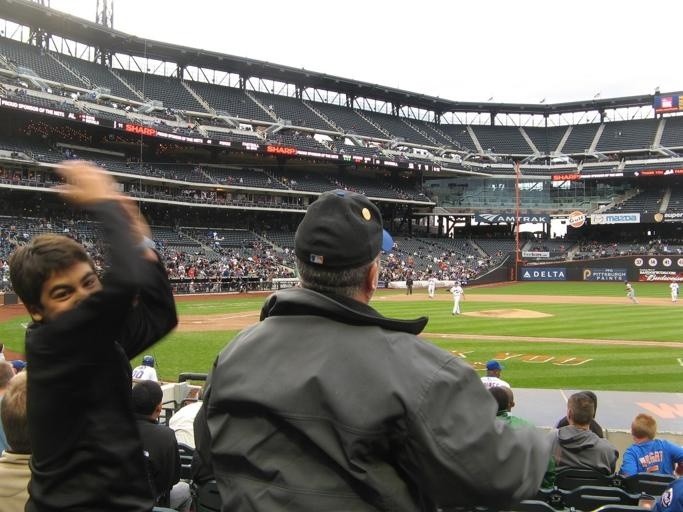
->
[11,359,25,369]
[487,361,504,372]
[291,186,394,272]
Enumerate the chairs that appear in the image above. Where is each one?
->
[135,402,682,512]
[0,34,683,286]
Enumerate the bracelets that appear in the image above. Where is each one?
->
[134,235,159,259]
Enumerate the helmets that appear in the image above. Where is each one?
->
[142,356,155,367]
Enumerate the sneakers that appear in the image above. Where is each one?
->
[429,295,433,298]
[672,300,677,303]
[452,312,460,315]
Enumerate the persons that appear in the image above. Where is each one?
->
[529,232,683,259]
[193,188,553,509]
[449,281,465,314]
[624,281,639,303]
[405,277,413,296]
[6,161,182,511]
[427,273,437,298]
[669,279,678,302]
